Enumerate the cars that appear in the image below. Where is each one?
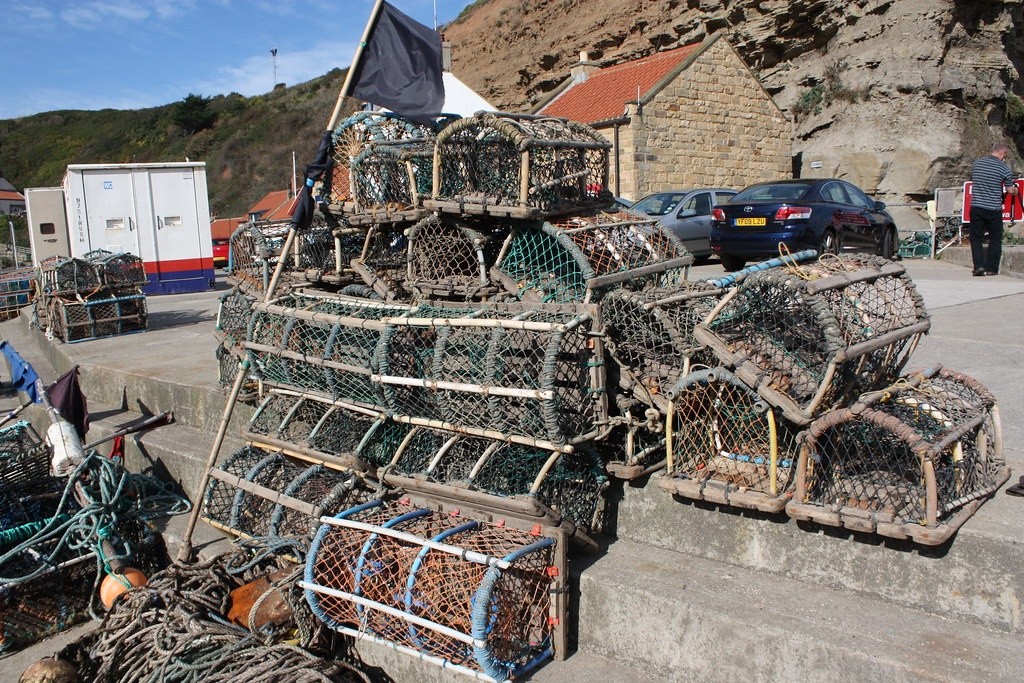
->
[628,188,742,262]
[709,175,899,270]
[211,238,234,268]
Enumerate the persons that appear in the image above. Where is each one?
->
[969,144,1019,277]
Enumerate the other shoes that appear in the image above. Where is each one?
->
[973,267,985,275]
[987,271,999,275]
